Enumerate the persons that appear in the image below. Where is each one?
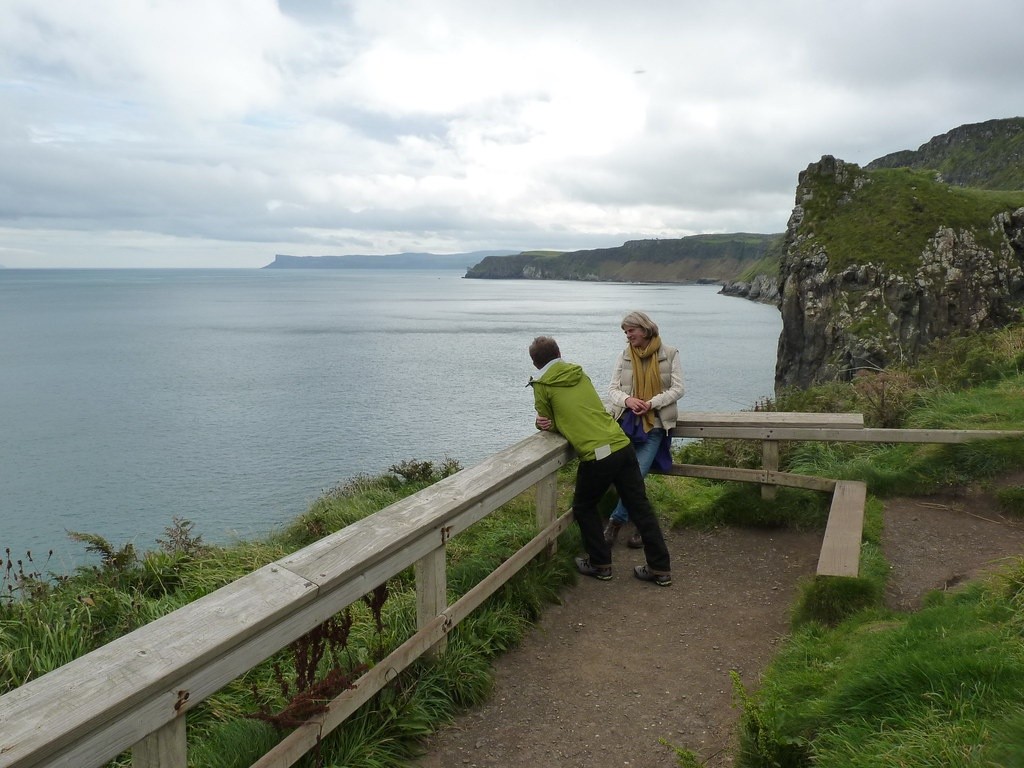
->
[525,336,672,586]
[604,312,685,549]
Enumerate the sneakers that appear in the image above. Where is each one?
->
[604,519,621,545]
[634,564,673,587]
[575,556,612,581]
[628,527,645,548]
